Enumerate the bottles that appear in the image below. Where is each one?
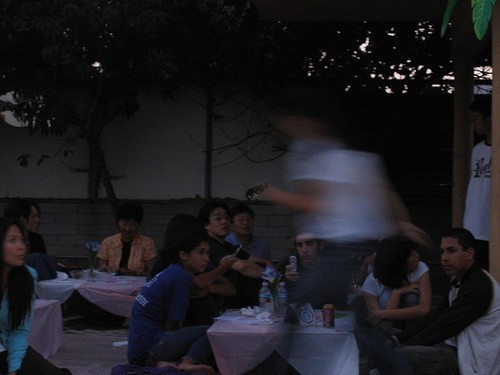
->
[276,282,288,323]
[259,281,274,311]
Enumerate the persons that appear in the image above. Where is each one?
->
[0,219,71,375]
[95,206,152,278]
[127,231,215,374]
[410,237,499,371]
[144,214,237,297]
[362,228,432,328]
[198,197,286,284]
[282,230,350,308]
[4,195,61,277]
[465,107,500,252]
[227,199,275,267]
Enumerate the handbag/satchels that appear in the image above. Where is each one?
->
[26,253,57,281]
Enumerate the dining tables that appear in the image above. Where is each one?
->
[206,308,360,375]
[37,276,148,329]
[28,300,64,359]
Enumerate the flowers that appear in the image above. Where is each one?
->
[84,240,101,274]
[261,264,286,289]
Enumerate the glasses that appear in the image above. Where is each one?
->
[3,238,27,245]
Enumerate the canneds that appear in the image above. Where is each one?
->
[323,304,335,328]
